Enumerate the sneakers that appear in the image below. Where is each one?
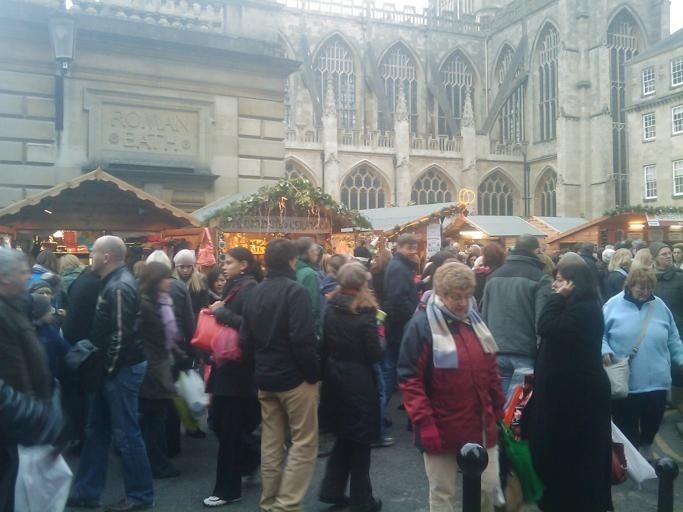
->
[202,495,243,507]
[241,470,262,488]
[187,427,207,439]
[370,435,394,448]
[65,493,102,510]
[319,495,350,504]
[348,499,383,512]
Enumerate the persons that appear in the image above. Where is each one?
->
[23,249,85,320]
[141,264,190,480]
[26,295,69,409]
[672,243,683,269]
[202,246,266,507]
[412,238,504,304]
[320,253,347,294]
[652,241,683,340]
[0,377,69,512]
[0,249,56,398]
[398,261,501,512]
[366,234,425,432]
[601,268,683,460]
[367,248,395,295]
[206,270,228,304]
[347,274,395,446]
[66,234,155,512]
[352,238,373,259]
[318,260,386,511]
[536,233,654,291]
[146,249,205,456]
[173,248,209,317]
[296,235,322,348]
[238,239,323,512]
[528,252,614,512]
[64,268,103,450]
[483,234,554,401]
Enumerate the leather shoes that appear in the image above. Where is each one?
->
[105,495,155,512]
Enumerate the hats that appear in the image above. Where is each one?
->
[602,249,616,264]
[173,248,196,266]
[649,241,669,257]
[336,263,372,288]
[144,250,171,269]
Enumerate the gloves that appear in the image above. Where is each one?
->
[419,426,442,456]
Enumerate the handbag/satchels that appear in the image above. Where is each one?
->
[612,442,629,486]
[494,444,524,511]
[191,283,240,353]
[497,418,545,500]
[502,374,536,440]
[604,303,654,400]
[196,227,216,268]
